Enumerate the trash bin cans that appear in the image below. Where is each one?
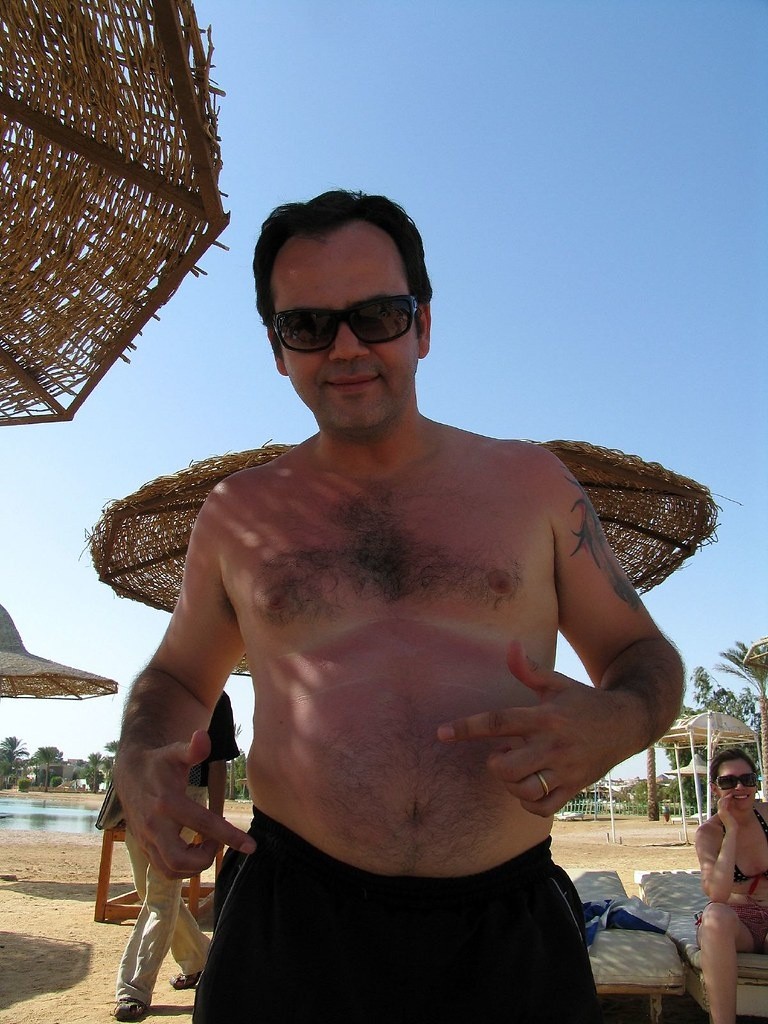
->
[7,784,15,789]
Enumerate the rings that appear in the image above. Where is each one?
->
[535,772,548,797]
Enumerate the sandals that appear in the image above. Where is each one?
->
[113,996,146,1021]
[170,969,203,990]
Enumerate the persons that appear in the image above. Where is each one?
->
[114,690,240,1021]
[663,803,670,822]
[696,748,768,1024]
[113,190,685,1024]
[609,797,616,813]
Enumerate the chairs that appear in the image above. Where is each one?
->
[671,812,707,825]
[553,812,584,822]
[564,868,768,1024]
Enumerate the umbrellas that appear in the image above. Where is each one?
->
[89,441,719,674]
[0,0,230,426]
[0,603,118,700]
[664,754,707,776]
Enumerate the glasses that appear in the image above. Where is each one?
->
[268,295,419,353]
[713,773,757,790]
